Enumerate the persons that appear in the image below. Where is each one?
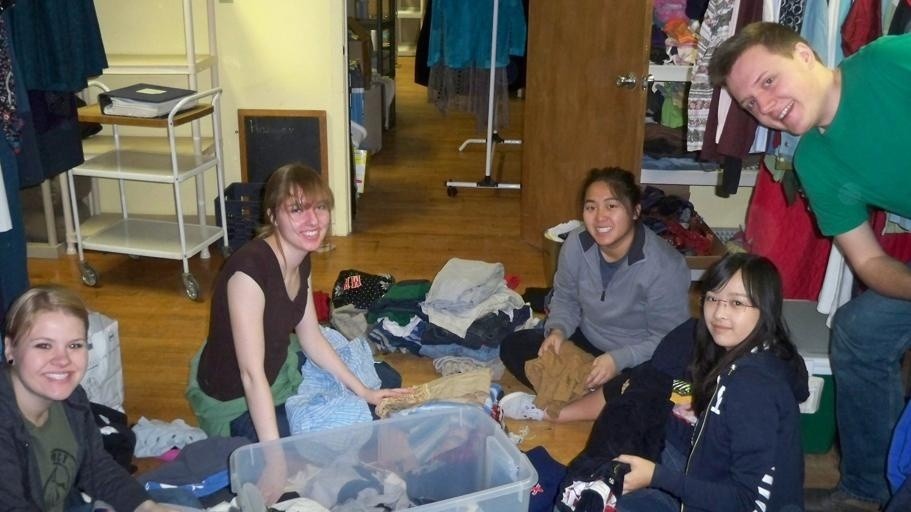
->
[499,165,692,429]
[549,252,807,511]
[706,21,911,512]
[0,283,205,512]
[181,162,416,509]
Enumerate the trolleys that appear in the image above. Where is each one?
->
[58,80,235,302]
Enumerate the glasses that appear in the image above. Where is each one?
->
[698,295,759,313]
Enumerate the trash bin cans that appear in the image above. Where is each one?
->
[541,222,583,288]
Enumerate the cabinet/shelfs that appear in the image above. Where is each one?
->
[358,1,397,134]
[58,1,227,260]
[641,64,793,282]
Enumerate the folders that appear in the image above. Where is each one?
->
[97,83,198,119]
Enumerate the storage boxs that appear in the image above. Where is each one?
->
[780,297,838,455]
[231,407,538,512]
[214,181,268,256]
